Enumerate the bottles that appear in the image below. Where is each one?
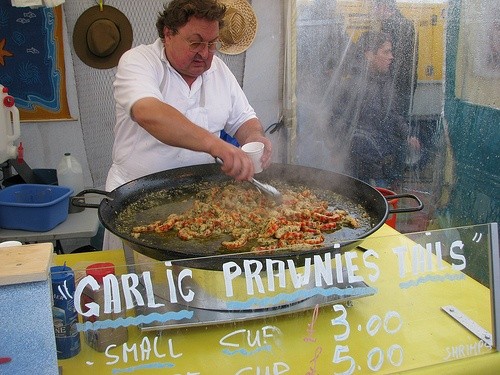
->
[50,266,80,360]
[79,263,128,354]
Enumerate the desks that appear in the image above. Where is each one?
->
[0,196,101,255]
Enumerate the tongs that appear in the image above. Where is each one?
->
[215,156,282,203]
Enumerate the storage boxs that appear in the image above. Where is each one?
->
[0,183,74,232]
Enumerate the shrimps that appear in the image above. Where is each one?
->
[131,184,358,254]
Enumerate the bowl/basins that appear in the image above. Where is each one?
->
[1,168,58,188]
[0,183,75,232]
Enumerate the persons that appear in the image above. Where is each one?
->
[102,0,272,250]
[332,30,421,187]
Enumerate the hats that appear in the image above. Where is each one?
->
[216,0,257,55]
[73,4,133,69]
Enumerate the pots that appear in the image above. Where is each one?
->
[70,161,424,272]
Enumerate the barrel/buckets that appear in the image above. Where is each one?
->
[375,187,398,229]
[0,83,22,177]
[56,152,83,196]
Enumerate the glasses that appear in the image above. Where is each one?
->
[175,29,223,52]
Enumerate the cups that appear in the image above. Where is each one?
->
[241,142,264,174]
